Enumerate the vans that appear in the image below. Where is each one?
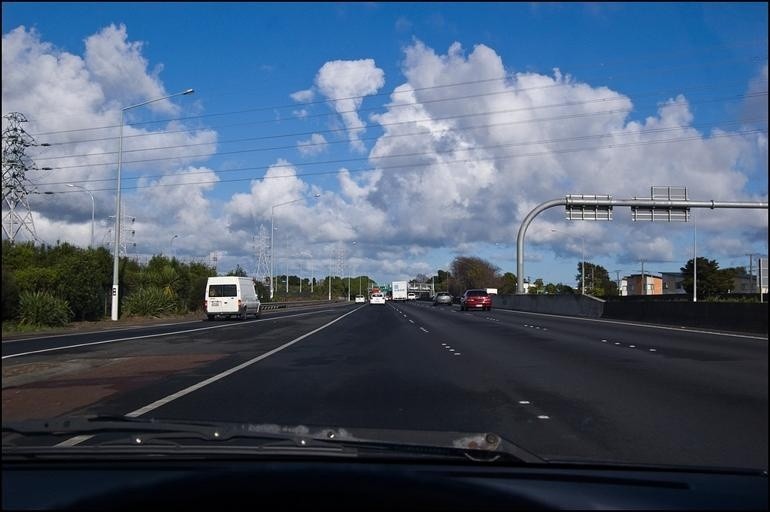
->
[201,275,264,320]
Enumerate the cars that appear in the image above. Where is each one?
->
[458,288,493,312]
[431,291,455,306]
[355,290,420,305]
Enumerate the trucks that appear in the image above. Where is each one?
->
[391,281,409,301]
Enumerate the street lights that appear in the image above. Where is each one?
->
[550,228,585,295]
[350,241,363,295]
[67,183,95,249]
[109,88,196,322]
[268,192,322,298]
[168,234,179,259]
[272,226,289,294]
